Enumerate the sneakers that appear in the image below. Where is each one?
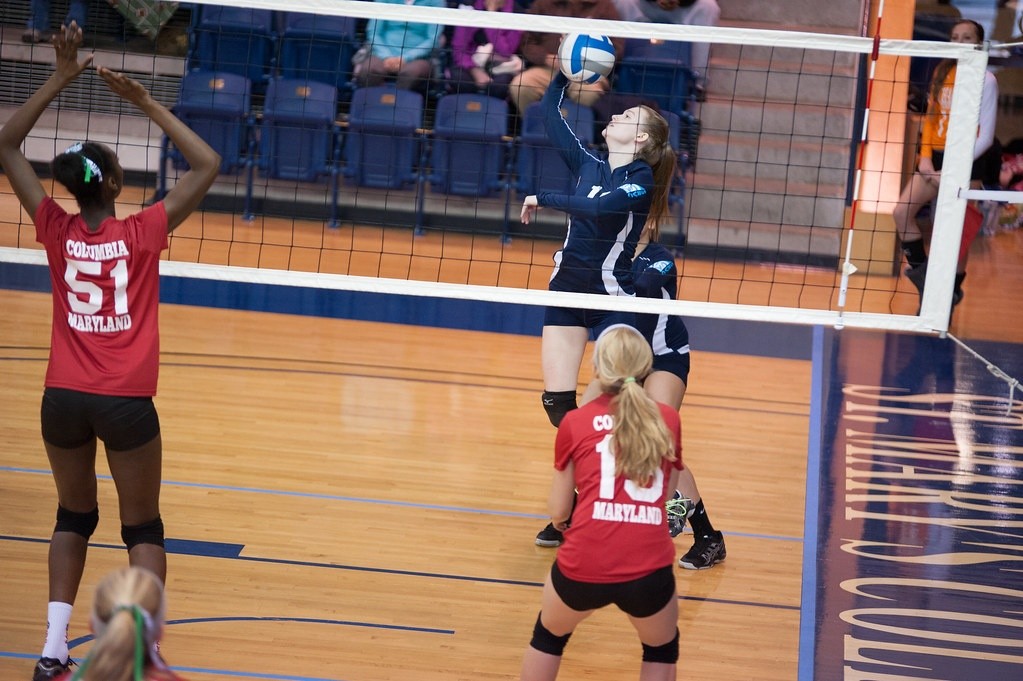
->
[678,527,726,571]
[33,654,79,681]
[666,492,693,538]
[536,514,569,547]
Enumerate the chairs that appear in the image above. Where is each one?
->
[141,3,712,258]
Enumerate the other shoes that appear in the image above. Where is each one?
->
[21,27,50,43]
[696,86,705,102]
[901,262,957,328]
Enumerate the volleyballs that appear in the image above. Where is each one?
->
[557,33,616,85]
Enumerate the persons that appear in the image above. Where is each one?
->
[21,0,89,46]
[1,20,223,680]
[895,19,1001,329]
[535,214,728,571]
[335,0,448,129]
[520,32,681,431]
[51,564,185,681]
[415,0,534,135]
[520,323,685,681]
[609,0,720,103]
[502,0,625,143]
